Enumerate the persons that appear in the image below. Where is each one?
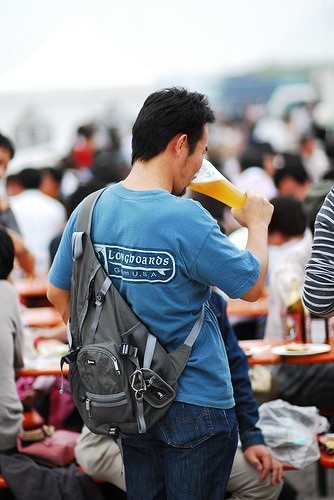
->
[45,86,275,500]
[0,99,334,341]
[73,217,285,500]
[0,232,25,456]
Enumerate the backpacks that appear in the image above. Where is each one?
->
[58,186,204,441]
